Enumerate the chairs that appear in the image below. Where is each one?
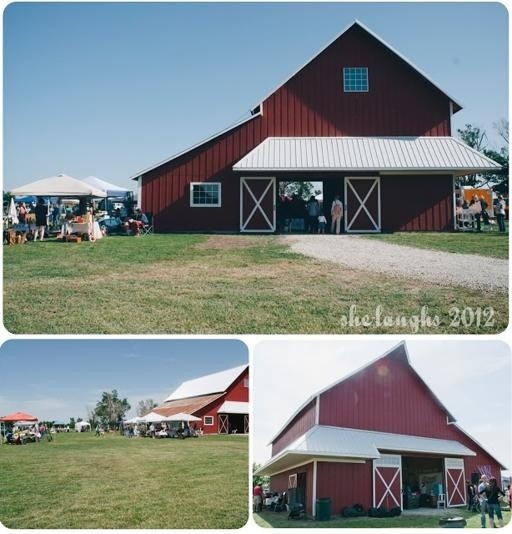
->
[437,494,445,510]
[140,212,153,238]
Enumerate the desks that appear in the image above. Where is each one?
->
[64,221,98,242]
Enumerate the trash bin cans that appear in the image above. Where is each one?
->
[316,497,333,520]
[439,516,466,528]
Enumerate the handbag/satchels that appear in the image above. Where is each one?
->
[334,201,341,215]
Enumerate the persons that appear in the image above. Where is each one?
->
[95,420,200,439]
[456,193,506,232]
[276,191,343,233]
[419,481,426,507]
[15,198,150,243]
[253,481,263,513]
[465,474,512,528]
[5,421,47,444]
